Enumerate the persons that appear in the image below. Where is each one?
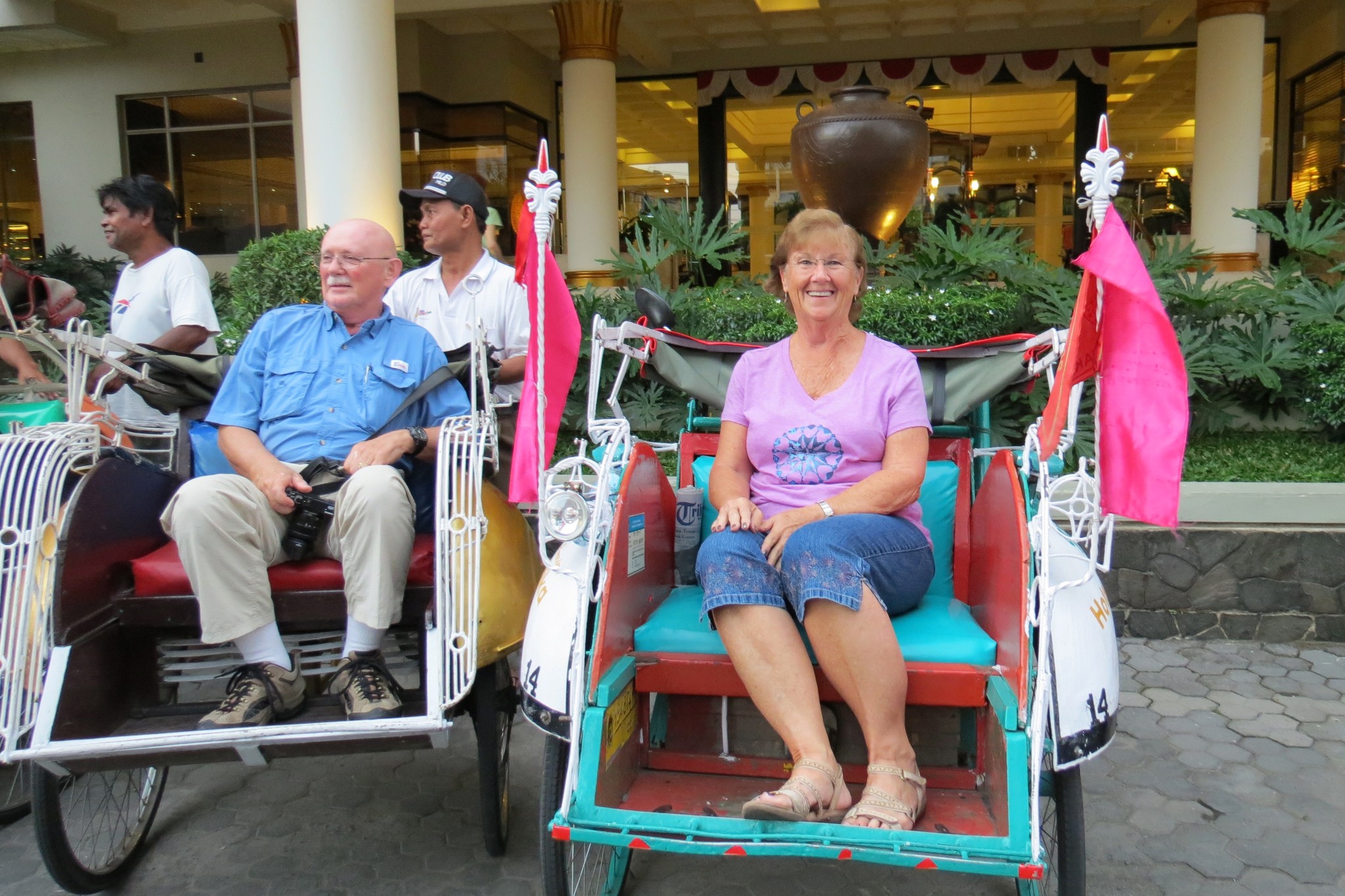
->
[692,207,936,833]
[0,166,531,730]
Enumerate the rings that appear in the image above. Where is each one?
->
[358,462,369,468]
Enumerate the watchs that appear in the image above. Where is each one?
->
[405,426,429,456]
[815,500,835,520]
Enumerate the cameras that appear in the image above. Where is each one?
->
[281,486,336,561]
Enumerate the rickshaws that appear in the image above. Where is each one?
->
[522,287,1117,896]
[0,311,528,895]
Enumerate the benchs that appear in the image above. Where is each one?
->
[634,434,997,791]
[110,404,435,718]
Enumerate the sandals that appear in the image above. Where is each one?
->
[843,764,928,829]
[742,761,851,824]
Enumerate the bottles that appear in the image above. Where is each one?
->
[573,467,622,547]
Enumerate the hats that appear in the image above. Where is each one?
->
[398,170,490,220]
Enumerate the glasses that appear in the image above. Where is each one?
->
[787,254,855,271]
[312,251,392,270]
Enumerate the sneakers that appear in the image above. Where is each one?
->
[199,648,307,731]
[326,646,410,721]
